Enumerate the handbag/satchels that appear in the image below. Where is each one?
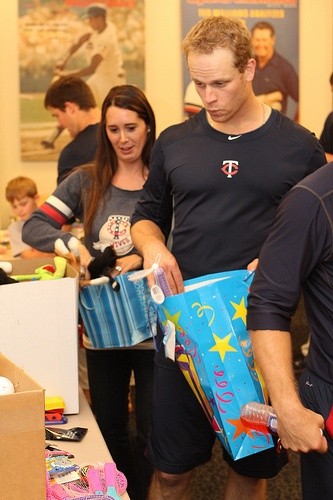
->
[78,270,158,350]
[147,269,276,462]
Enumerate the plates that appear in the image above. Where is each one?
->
[69,464,127,498]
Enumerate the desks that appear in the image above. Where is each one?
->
[46,384,130,500]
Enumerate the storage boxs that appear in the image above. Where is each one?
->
[0,256,79,414]
[0,353,47,500]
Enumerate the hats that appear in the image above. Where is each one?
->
[82,7,106,19]
[184,80,205,114]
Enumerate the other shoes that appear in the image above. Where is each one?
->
[42,140,53,148]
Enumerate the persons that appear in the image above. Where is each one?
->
[6,177,53,257]
[130,17,315,500]
[21,85,175,500]
[316,69,333,164]
[32,75,104,201]
[248,21,300,115]
[52,3,126,126]
[248,162,333,500]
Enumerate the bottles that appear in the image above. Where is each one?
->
[241,402,323,440]
